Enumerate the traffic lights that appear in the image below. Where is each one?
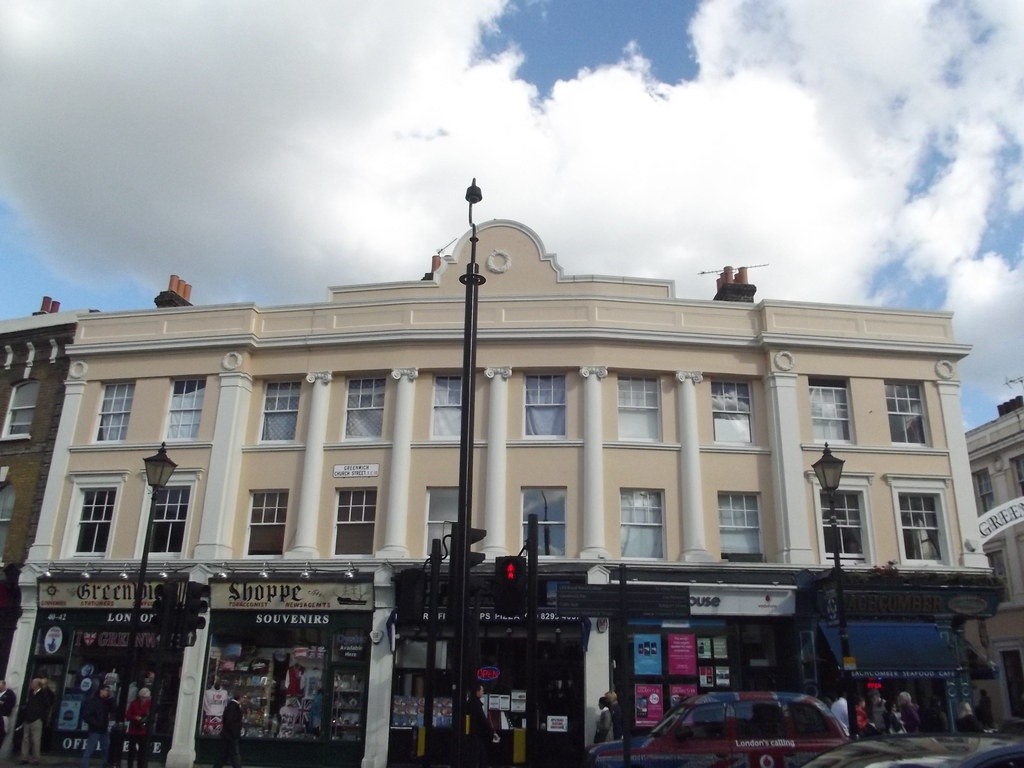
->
[183,581,212,630]
[493,555,526,617]
[448,523,485,604]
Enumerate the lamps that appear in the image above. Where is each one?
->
[119,562,131,581]
[44,561,57,577]
[218,562,230,581]
[300,562,315,580]
[343,561,359,581]
[258,561,270,580]
[159,562,170,580]
[80,562,93,581]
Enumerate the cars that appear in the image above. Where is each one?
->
[798,732,1024,768]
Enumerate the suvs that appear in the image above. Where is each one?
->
[589,690,847,768]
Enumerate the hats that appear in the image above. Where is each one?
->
[138,688,151,697]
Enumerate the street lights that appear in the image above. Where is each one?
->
[104,441,178,768]
[448,179,486,768]
[810,442,859,739]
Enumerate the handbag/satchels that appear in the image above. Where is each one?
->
[593,728,608,744]
[888,714,907,734]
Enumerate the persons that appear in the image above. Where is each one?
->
[80,686,117,768]
[127,688,152,768]
[15,678,49,765]
[596,697,614,742]
[957,702,984,732]
[214,690,244,768]
[0,680,17,747]
[979,689,994,724]
[466,683,491,768]
[605,691,623,740]
[831,690,949,738]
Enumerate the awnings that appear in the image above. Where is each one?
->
[819,622,961,680]
